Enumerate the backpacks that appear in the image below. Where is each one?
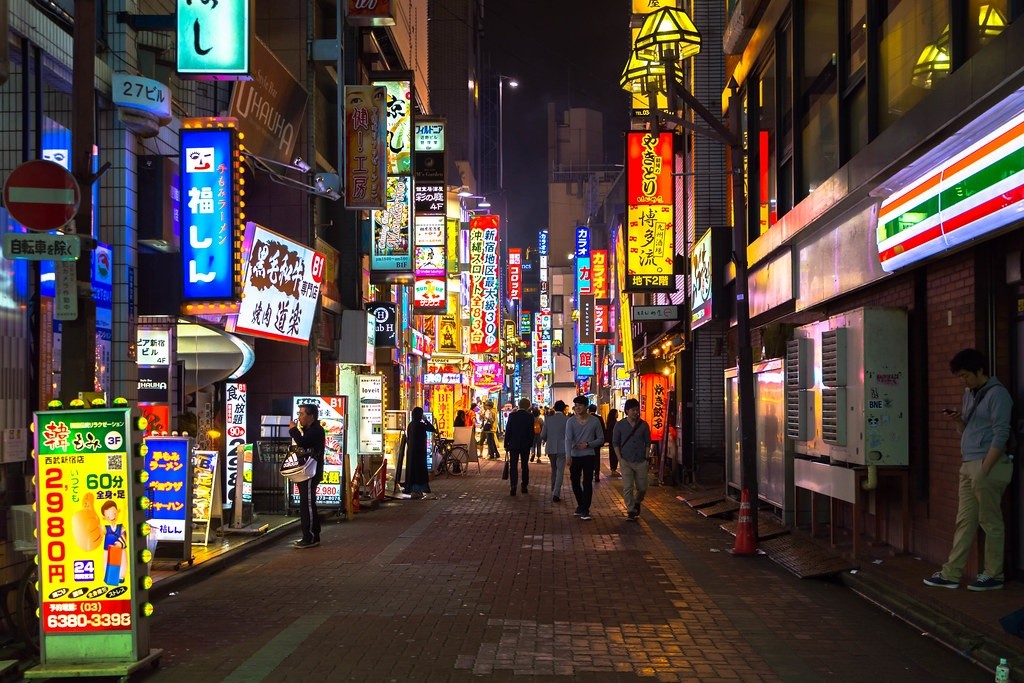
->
[533,417,541,434]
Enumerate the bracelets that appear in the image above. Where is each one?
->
[586,442,589,448]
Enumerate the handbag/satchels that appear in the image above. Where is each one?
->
[502,452,510,480]
[279,444,319,483]
[483,421,492,431]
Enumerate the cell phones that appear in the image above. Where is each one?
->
[942,409,957,414]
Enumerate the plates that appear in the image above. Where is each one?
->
[316,419,344,466]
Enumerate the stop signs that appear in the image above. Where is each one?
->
[2,159,84,234]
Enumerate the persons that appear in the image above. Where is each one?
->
[289,404,326,549]
[564,395,605,520]
[923,350,1015,592]
[452,399,605,500]
[612,398,652,521]
[403,407,436,498]
[345,86,383,114]
[606,409,622,476]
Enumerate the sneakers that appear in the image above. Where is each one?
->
[923,570,960,589]
[967,570,1004,592]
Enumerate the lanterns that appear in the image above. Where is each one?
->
[638,373,671,441]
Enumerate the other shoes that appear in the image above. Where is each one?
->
[294,539,320,549]
[553,496,559,502]
[626,514,635,520]
[530,453,535,462]
[595,474,600,482]
[537,460,541,463]
[574,510,581,516]
[580,515,592,519]
[633,506,641,516]
[611,470,622,476]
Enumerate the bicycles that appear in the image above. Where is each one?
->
[431,436,470,476]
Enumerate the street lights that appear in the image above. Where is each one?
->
[617,6,760,560]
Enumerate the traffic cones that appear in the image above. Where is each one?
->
[344,463,366,513]
[728,488,766,557]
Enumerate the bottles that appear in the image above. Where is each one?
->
[996,658,1009,683]
